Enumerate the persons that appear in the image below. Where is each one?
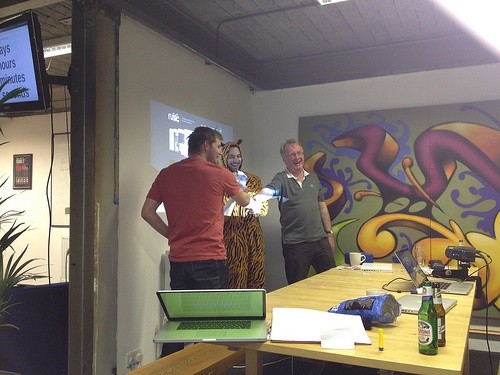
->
[219,137,269,289]
[250,139,336,285]
[140,126,262,358]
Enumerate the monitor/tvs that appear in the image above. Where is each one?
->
[0,9,50,113]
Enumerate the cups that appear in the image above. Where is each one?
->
[414,244,425,267]
[349,252,366,266]
[366,290,387,296]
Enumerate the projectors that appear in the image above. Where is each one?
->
[446,246,475,262]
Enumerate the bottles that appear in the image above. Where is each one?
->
[432,281,445,347]
[457,240,465,269]
[418,280,438,355]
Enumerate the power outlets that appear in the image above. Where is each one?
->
[125,348,142,368]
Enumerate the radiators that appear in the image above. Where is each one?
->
[60,237,70,282]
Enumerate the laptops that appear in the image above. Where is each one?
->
[395,247,473,294]
[361,263,392,272]
[396,295,457,314]
[153,289,267,342]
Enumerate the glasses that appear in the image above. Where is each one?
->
[285,151,304,157]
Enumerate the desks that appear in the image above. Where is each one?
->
[194,262,478,375]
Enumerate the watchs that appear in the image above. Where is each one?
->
[326,230,334,234]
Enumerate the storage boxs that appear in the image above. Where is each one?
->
[345,253,373,264]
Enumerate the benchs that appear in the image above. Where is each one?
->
[127,343,245,375]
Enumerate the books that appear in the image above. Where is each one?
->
[361,263,393,272]
[269,307,372,349]
